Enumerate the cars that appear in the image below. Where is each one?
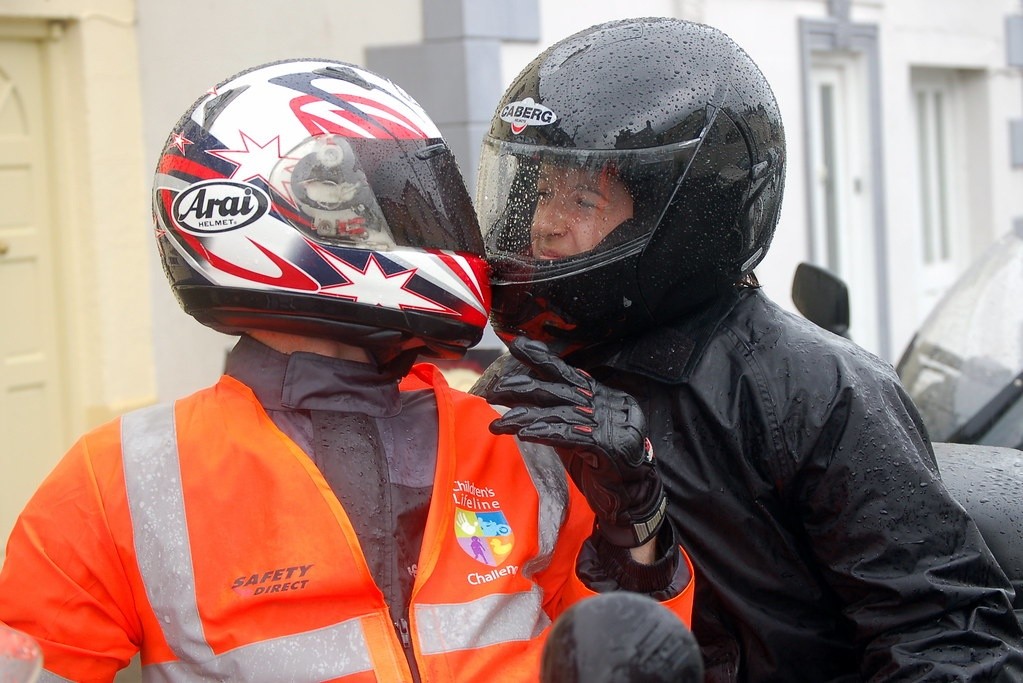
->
[791,218,1022,598]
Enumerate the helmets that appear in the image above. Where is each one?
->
[476,16,790,368]
[152,58,494,357]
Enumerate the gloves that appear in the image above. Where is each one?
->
[486,337,670,550]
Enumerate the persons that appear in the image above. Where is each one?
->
[0,58,695,683]
[476,17,1022,683]
